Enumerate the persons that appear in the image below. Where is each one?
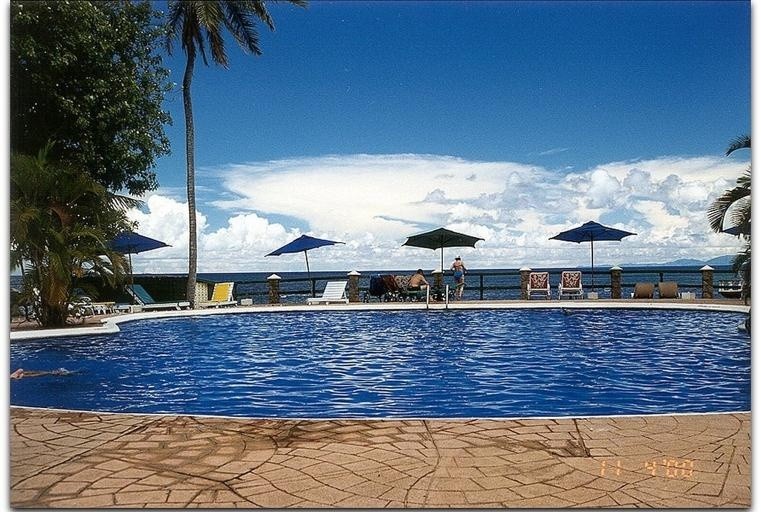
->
[407,268,436,302]
[449,254,467,301]
[11,366,81,382]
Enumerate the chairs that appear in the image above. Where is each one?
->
[633,282,679,299]
[363,275,444,303]
[307,281,350,304]
[80,282,239,316]
[526,270,584,301]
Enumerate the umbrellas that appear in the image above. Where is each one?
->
[547,221,637,292]
[401,227,485,287]
[90,228,174,303]
[264,234,346,299]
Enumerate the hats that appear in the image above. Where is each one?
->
[455,256,460,258]
